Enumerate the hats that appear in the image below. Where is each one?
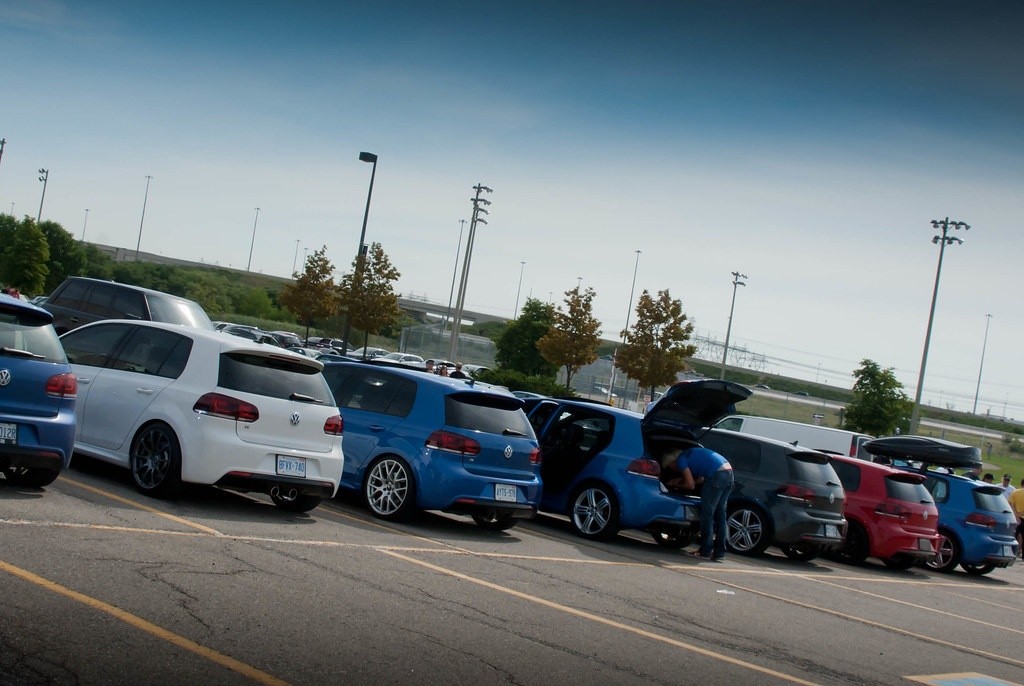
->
[456,362,462,368]
[984,473,994,479]
[1003,474,1011,481]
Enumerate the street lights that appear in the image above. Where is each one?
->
[623,250,643,343]
[910,218,971,436]
[973,313,992,417]
[38,167,49,224]
[720,272,747,378]
[135,175,153,261]
[445,219,468,329]
[247,207,261,272]
[340,152,377,356]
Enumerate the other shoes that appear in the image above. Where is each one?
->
[710,551,725,562]
[685,550,711,559]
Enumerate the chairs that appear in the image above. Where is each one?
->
[148,346,179,375]
[550,424,616,458]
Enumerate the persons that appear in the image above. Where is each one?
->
[935,467,1024,560]
[1,284,19,299]
[439,365,449,377]
[662,448,734,563]
[426,360,435,373]
[450,362,465,379]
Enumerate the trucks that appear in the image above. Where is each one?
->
[716,415,874,461]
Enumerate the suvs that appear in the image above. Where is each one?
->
[862,436,1020,576]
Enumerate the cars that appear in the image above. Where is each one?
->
[694,426,849,561]
[828,453,941,571]
[323,362,543,529]
[0,292,77,487]
[29,276,493,377]
[58,319,345,512]
[523,380,754,549]
